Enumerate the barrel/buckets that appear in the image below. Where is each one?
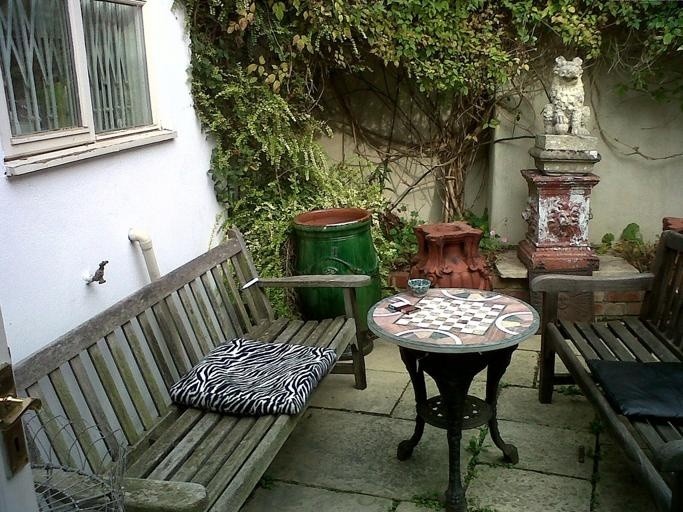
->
[292,208,383,362]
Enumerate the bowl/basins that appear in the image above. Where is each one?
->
[407,278,430,298]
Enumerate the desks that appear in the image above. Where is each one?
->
[365,286,539,510]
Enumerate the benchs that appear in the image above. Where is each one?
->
[7,224,373,510]
[529,230,681,512]
